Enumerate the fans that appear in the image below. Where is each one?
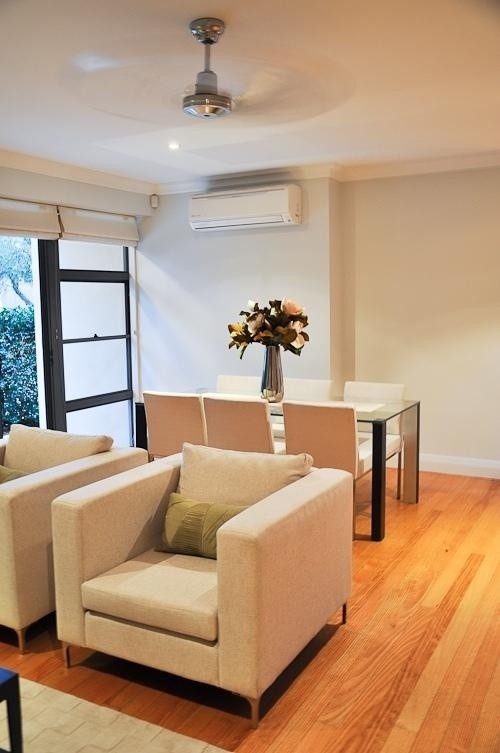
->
[64,13,359,132]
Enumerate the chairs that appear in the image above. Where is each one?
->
[140,389,207,465]
[215,375,261,395]
[201,392,286,458]
[0,420,152,656]
[271,376,334,439]
[282,401,373,543]
[49,439,359,729]
[342,380,408,502]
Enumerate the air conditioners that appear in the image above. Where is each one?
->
[187,181,301,232]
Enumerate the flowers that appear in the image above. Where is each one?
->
[222,295,314,361]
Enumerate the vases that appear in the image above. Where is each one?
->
[258,343,290,405]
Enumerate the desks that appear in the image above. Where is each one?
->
[0,667,32,752]
[130,390,421,544]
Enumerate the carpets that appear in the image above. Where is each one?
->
[0,675,233,753]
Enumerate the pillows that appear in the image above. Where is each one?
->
[151,487,251,559]
[0,462,28,484]
